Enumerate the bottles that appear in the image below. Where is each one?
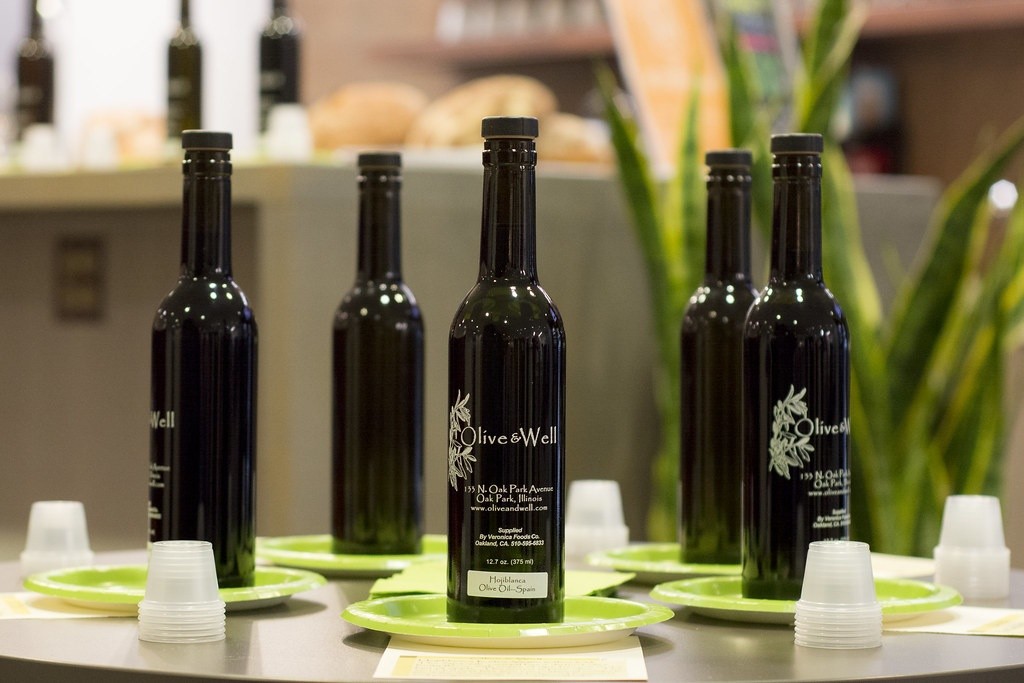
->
[167,0,202,138]
[742,131,852,601]
[15,1,55,141]
[260,1,298,133]
[329,151,428,556]
[147,129,261,590]
[448,114,566,624]
[678,149,757,565]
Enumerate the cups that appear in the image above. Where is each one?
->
[18,478,1015,655]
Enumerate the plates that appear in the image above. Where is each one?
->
[341,592,675,651]
[256,535,447,580]
[22,561,325,613]
[648,576,964,626]
[586,540,745,587]
